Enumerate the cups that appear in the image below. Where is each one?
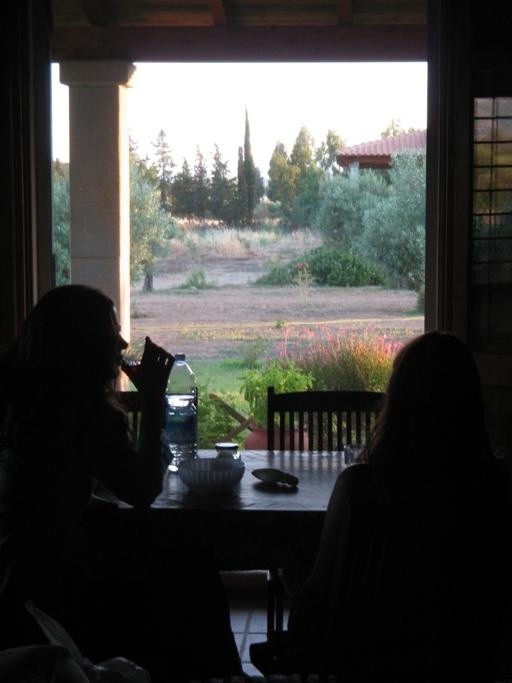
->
[120,337,147,366]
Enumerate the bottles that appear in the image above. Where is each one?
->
[163,352,198,474]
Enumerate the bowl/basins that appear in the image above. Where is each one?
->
[179,458,247,489]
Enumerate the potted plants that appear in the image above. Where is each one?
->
[239,366,317,450]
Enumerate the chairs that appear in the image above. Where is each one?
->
[267,386,384,640]
[0,645,92,683]
[116,391,199,455]
[252,497,512,683]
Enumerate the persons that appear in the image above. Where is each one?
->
[1,284,270,683]
[287,328,512,681]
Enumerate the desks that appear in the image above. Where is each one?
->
[151,450,384,515]
